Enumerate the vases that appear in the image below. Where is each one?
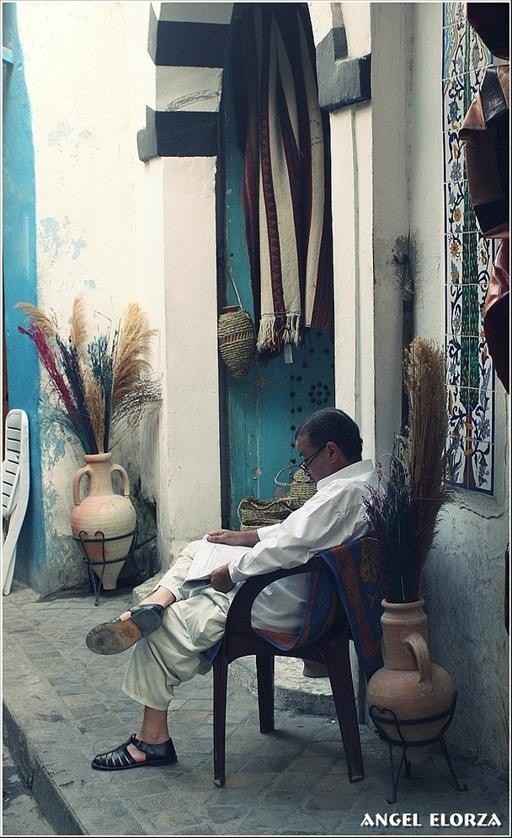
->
[72,452,139,590]
[364,598,458,742]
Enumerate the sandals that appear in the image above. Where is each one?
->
[91,734,177,770]
[86,604,164,655]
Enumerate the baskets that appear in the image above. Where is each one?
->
[237,468,317,531]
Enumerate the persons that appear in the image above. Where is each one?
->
[84,405,389,773]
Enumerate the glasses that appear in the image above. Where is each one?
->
[299,446,325,472]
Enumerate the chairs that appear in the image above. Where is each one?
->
[213,532,388,787]
